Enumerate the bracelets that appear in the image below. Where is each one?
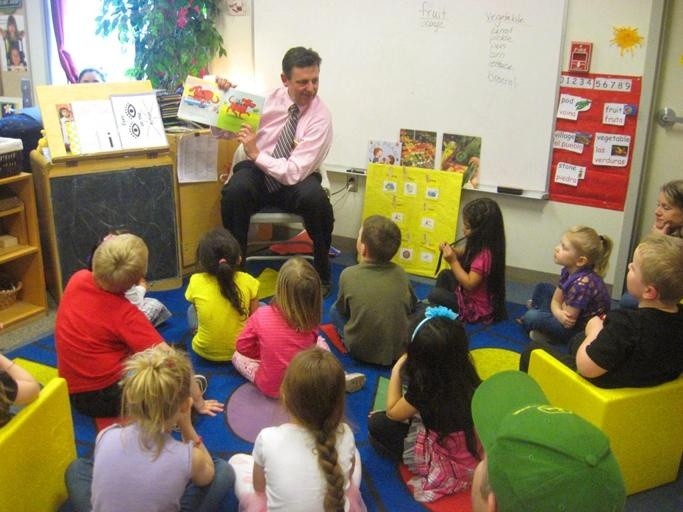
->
[191,435,204,448]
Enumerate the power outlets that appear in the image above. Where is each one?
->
[346,172,358,195]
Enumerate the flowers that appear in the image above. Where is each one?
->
[94,0,228,89]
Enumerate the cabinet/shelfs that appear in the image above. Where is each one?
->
[0,172,47,342]
[163,125,239,279]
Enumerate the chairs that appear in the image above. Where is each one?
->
[1,356,76,512]
[225,134,328,272]
[526,347,682,501]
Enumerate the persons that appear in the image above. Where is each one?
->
[101,229,173,329]
[4,15,27,71]
[516,180,683,389]
[64,347,216,511]
[373,148,383,163]
[383,155,394,164]
[231,257,366,399]
[215,45,333,298]
[0,353,39,427]
[53,235,225,417]
[368,305,484,503]
[428,198,507,323]
[79,68,104,83]
[228,347,368,512]
[183,229,263,366]
[60,107,73,153]
[336,214,423,367]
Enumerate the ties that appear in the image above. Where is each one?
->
[265,105,299,192]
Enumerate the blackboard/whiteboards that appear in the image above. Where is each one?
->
[251,0,569,200]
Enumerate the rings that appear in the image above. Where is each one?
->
[244,134,247,137]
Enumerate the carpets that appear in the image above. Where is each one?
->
[0,262,682,512]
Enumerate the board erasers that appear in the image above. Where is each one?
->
[497,187,523,195]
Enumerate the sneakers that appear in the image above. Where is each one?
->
[529,329,552,343]
[320,261,333,298]
[344,372,366,393]
[194,375,207,396]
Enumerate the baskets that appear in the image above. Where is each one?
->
[0,150,21,178]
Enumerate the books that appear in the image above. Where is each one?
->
[176,76,265,135]
[157,93,203,128]
